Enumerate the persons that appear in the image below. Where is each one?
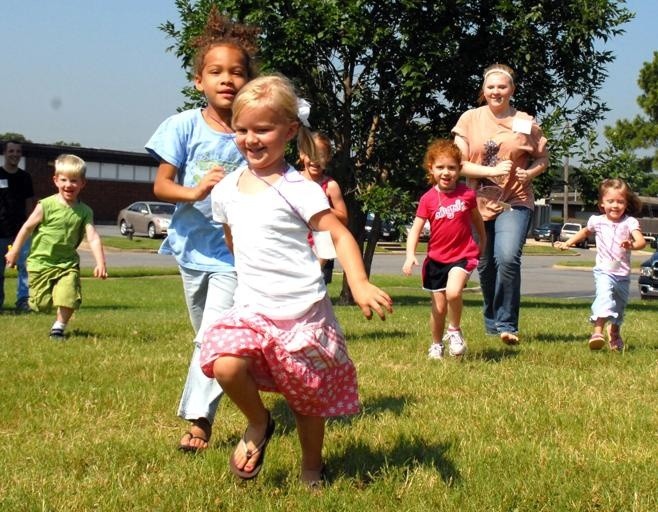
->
[449,64,551,345]
[404,137,488,361]
[557,178,647,349]
[199,74,394,491]
[1,140,33,315]
[297,133,348,272]
[5,152,107,338]
[143,0,260,454]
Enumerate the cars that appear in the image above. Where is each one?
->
[639,249,657,298]
[533,223,595,249]
[118,202,175,239]
[363,215,430,241]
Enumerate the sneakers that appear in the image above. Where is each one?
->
[589,333,624,350]
[50,329,63,337]
[428,327,466,359]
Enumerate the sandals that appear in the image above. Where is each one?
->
[229,408,275,479]
[178,431,207,452]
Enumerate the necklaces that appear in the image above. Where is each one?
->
[436,189,449,208]
